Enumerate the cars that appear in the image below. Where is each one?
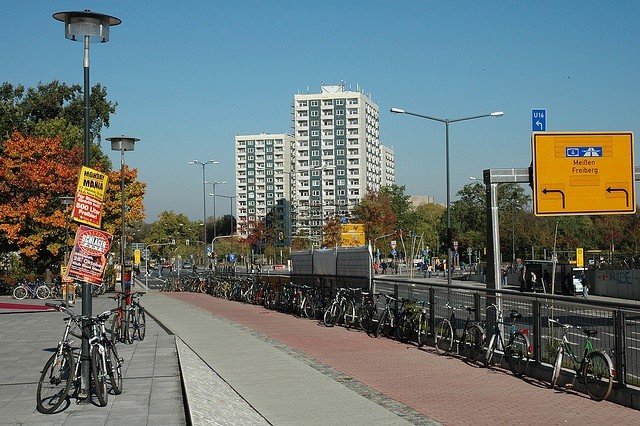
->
[183,261,191,268]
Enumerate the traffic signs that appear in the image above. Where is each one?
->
[533,132,637,216]
[531,109,546,132]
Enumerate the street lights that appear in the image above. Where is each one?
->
[189,159,217,270]
[204,181,227,268]
[58,195,75,308]
[105,135,140,341]
[209,193,239,268]
[53,9,123,404]
[390,107,504,339]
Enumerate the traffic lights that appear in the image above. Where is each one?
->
[335,210,348,213]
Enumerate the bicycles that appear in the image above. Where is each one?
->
[108,292,122,343]
[376,293,409,338]
[37,303,122,414]
[117,292,146,344]
[324,287,346,326]
[344,287,380,332]
[435,302,486,361]
[484,302,532,375]
[157,274,325,319]
[397,301,434,346]
[13,279,50,300]
[49,279,105,298]
[548,318,615,401]
[63,315,123,406]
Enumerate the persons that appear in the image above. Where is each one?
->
[580,274,590,300]
[530,271,536,293]
[373,262,378,274]
[415,262,439,278]
[502,266,510,286]
[192,264,197,273]
[390,261,398,274]
[518,267,527,292]
[450,262,455,276]
[459,262,466,274]
[170,263,177,275]
[380,261,387,274]
